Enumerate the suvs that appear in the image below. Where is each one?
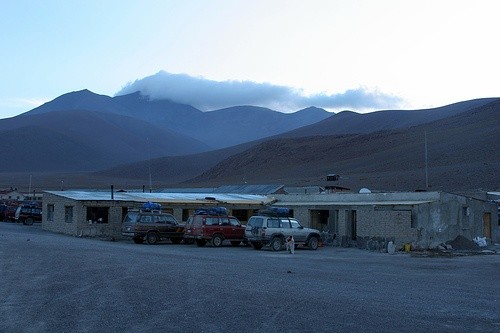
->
[14,205,42,225]
[246,216,321,251]
[184,214,250,248]
[120,208,188,245]
[0,202,18,223]
